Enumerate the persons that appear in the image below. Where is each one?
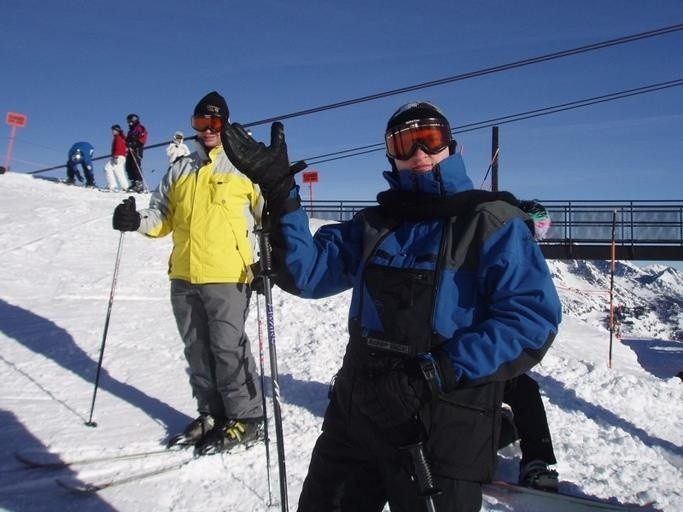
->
[102,124,128,191]
[65,142,97,187]
[166,131,190,167]
[123,114,147,193]
[110,89,268,458]
[218,102,561,512]
[497,372,559,494]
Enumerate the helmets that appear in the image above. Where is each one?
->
[71,150,82,164]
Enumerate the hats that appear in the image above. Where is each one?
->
[381,100,454,146]
[126,113,140,125]
[191,88,232,121]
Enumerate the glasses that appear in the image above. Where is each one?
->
[176,136,182,139]
[190,116,226,133]
[383,116,453,161]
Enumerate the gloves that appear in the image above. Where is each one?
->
[218,120,308,205]
[111,196,142,233]
[247,258,277,297]
[113,157,118,166]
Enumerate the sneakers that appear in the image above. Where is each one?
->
[167,412,262,456]
[63,173,145,194]
[517,455,561,493]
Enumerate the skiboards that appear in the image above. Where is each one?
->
[483,481,662,511]
[15,416,270,493]
